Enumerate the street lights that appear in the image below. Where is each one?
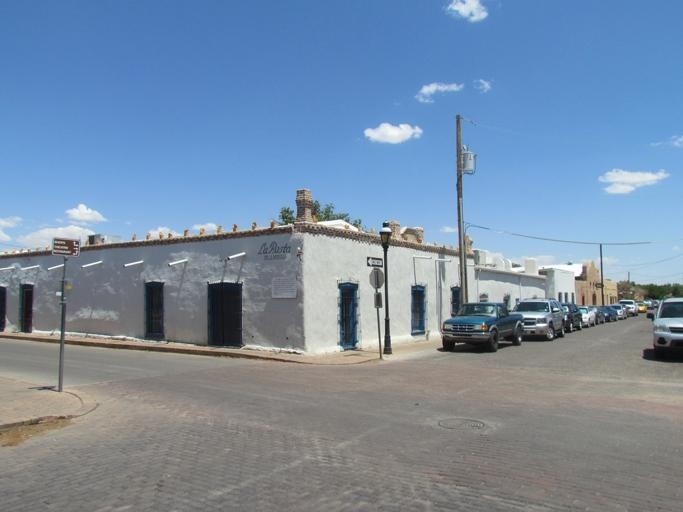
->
[378,220,395,352]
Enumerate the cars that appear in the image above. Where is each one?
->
[513,297,659,351]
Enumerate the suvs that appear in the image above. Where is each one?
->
[645,297,683,354]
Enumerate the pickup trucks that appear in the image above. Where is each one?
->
[440,302,525,354]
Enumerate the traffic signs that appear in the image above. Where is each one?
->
[51,238,80,257]
[366,257,383,268]
